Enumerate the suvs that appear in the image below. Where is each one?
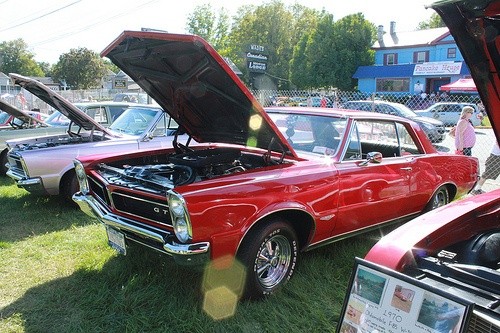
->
[413,102,484,128]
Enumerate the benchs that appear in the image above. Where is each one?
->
[331,139,421,159]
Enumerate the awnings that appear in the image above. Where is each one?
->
[353,61,471,78]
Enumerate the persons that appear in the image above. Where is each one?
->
[320,97,327,107]
[471,139,500,195]
[130,96,138,103]
[421,90,447,100]
[475,97,486,117]
[88,96,93,102]
[306,96,313,107]
[455,106,476,155]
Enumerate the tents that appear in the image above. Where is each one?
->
[440,78,477,91]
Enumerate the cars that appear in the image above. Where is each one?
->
[0,72,198,210]
[342,99,447,143]
[291,96,347,109]
[361,0,500,333]
[72,29,481,303]
